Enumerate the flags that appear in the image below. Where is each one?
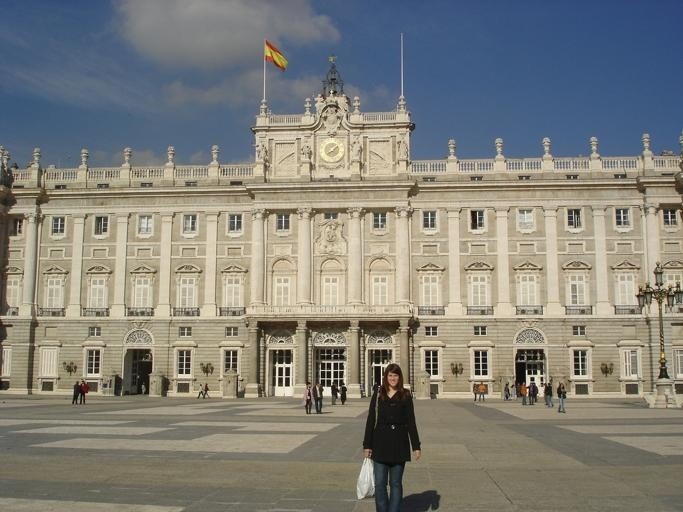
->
[264,39,289,72]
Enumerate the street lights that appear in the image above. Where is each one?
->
[635,259,682,409]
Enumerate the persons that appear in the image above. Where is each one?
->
[473,383,478,401]
[303,381,313,415]
[478,382,486,401]
[362,363,421,512]
[330,381,339,405]
[339,382,348,405]
[503,381,567,413]
[202,384,210,399]
[312,381,325,414]
[79,380,88,404]
[72,381,79,407]
[197,384,205,399]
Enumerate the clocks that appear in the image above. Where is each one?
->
[319,137,344,163]
